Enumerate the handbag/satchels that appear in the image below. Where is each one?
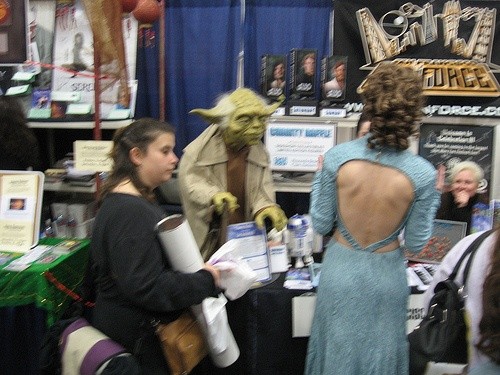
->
[414,229,496,364]
[155,313,209,375]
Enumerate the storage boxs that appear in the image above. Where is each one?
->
[67,217,96,239]
[52,198,80,220]
[52,220,68,238]
[69,199,96,223]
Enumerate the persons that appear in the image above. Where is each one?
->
[322,63,345,99]
[296,63,445,374]
[296,53,315,91]
[85,116,226,375]
[266,63,285,98]
[420,225,500,375]
[440,160,486,239]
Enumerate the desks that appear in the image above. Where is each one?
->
[0,239,91,375]
[199,262,436,375]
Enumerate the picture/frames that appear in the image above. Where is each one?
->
[405,219,467,265]
[408,117,500,204]
